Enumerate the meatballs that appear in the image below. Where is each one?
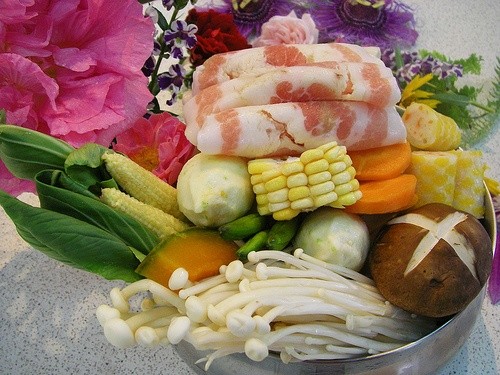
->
[292,209,369,272]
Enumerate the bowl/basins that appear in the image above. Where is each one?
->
[148,105,497,374]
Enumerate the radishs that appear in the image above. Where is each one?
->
[176,153,254,230]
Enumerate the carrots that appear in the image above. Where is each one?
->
[344,142,420,218]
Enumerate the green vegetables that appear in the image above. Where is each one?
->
[0,124,160,283]
[393,46,496,132]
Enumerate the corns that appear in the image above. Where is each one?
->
[96,151,190,242]
[248,141,363,222]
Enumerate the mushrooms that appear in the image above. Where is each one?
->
[369,202,493,318]
